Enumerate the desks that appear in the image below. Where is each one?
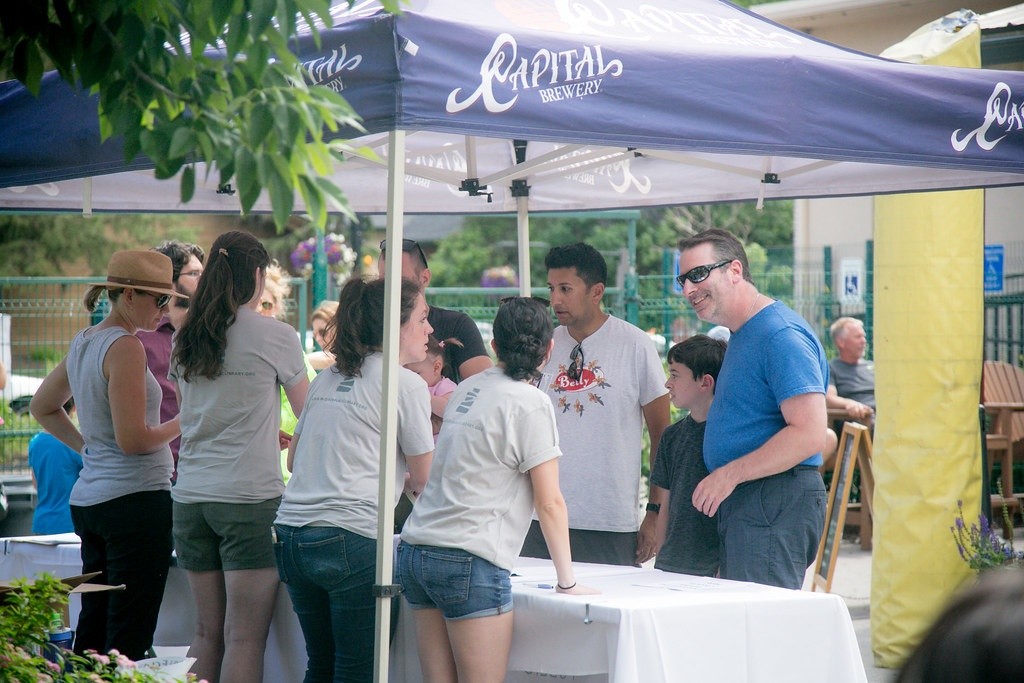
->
[0,536,868,683]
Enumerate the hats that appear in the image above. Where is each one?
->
[87,250,190,299]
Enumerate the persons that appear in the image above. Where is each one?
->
[0,229,873,589]
[273,274,435,683]
[30,248,181,661]
[396,295,606,683]
[897,566,1024,683]
[167,233,310,683]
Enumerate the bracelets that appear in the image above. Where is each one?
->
[558,582,576,590]
[646,501,661,513]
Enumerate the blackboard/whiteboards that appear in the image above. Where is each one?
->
[813,421,875,591]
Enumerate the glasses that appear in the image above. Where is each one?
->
[499,296,552,317]
[262,301,276,311]
[313,328,329,338]
[380,239,428,268]
[141,291,173,309]
[676,259,733,288]
[178,269,203,277]
[568,343,584,380]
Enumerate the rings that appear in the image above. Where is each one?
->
[654,553,657,555]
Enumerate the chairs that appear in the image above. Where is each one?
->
[825,361,1024,549]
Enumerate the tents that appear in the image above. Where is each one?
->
[0,0,1024,683]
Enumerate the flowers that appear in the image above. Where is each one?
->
[290,231,357,279]
[481,265,517,287]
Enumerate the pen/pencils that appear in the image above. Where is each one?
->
[522,583,554,589]
